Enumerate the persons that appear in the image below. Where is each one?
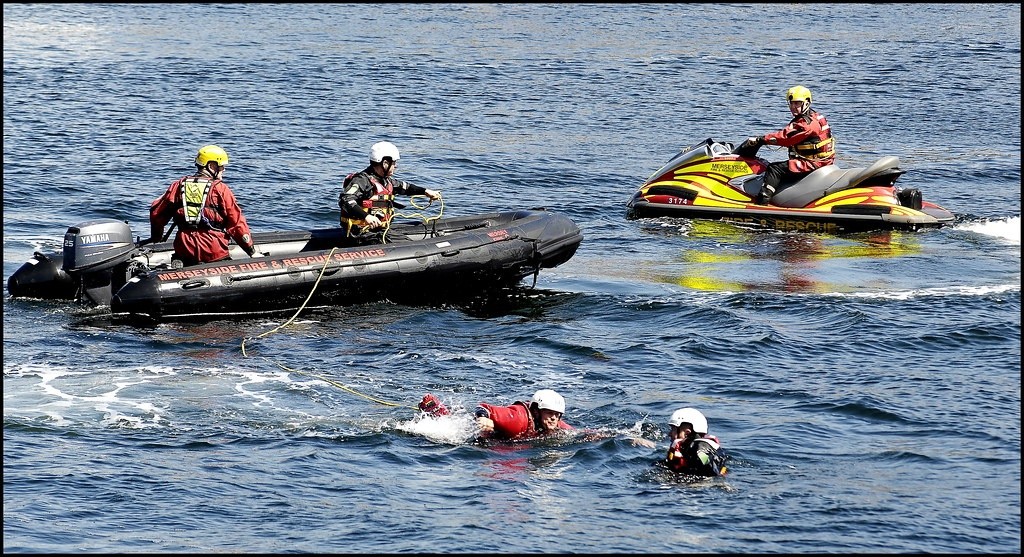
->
[419,389,654,447]
[748,84,836,206]
[337,142,441,247]
[150,146,265,268]
[659,407,730,476]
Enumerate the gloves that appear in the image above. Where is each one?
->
[747,137,759,146]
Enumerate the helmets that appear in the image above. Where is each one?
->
[195,145,229,167]
[369,141,400,163]
[787,85,812,104]
[529,389,566,414]
[667,408,708,434]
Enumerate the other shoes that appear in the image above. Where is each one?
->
[418,395,435,410]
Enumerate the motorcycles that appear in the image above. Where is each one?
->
[625,135,960,231]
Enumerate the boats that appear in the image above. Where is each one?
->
[8,190,584,325]
